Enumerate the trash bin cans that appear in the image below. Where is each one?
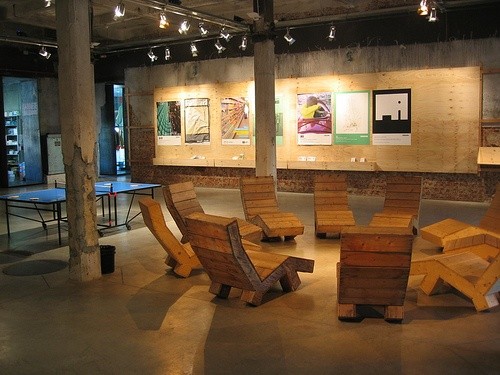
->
[99,245,117,274]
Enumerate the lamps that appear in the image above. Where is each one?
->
[39,0,337,60]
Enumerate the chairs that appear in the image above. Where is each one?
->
[337,227,413,320]
[239,176,304,240]
[369,176,423,236]
[410,247,500,311]
[421,192,500,250]
[184,212,314,306]
[162,181,262,248]
[313,174,355,239]
[138,197,201,278]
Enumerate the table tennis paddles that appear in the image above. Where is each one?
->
[29,198,39,201]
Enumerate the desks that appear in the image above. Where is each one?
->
[0,180,159,245]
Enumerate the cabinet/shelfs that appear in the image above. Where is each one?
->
[5,115,26,184]
